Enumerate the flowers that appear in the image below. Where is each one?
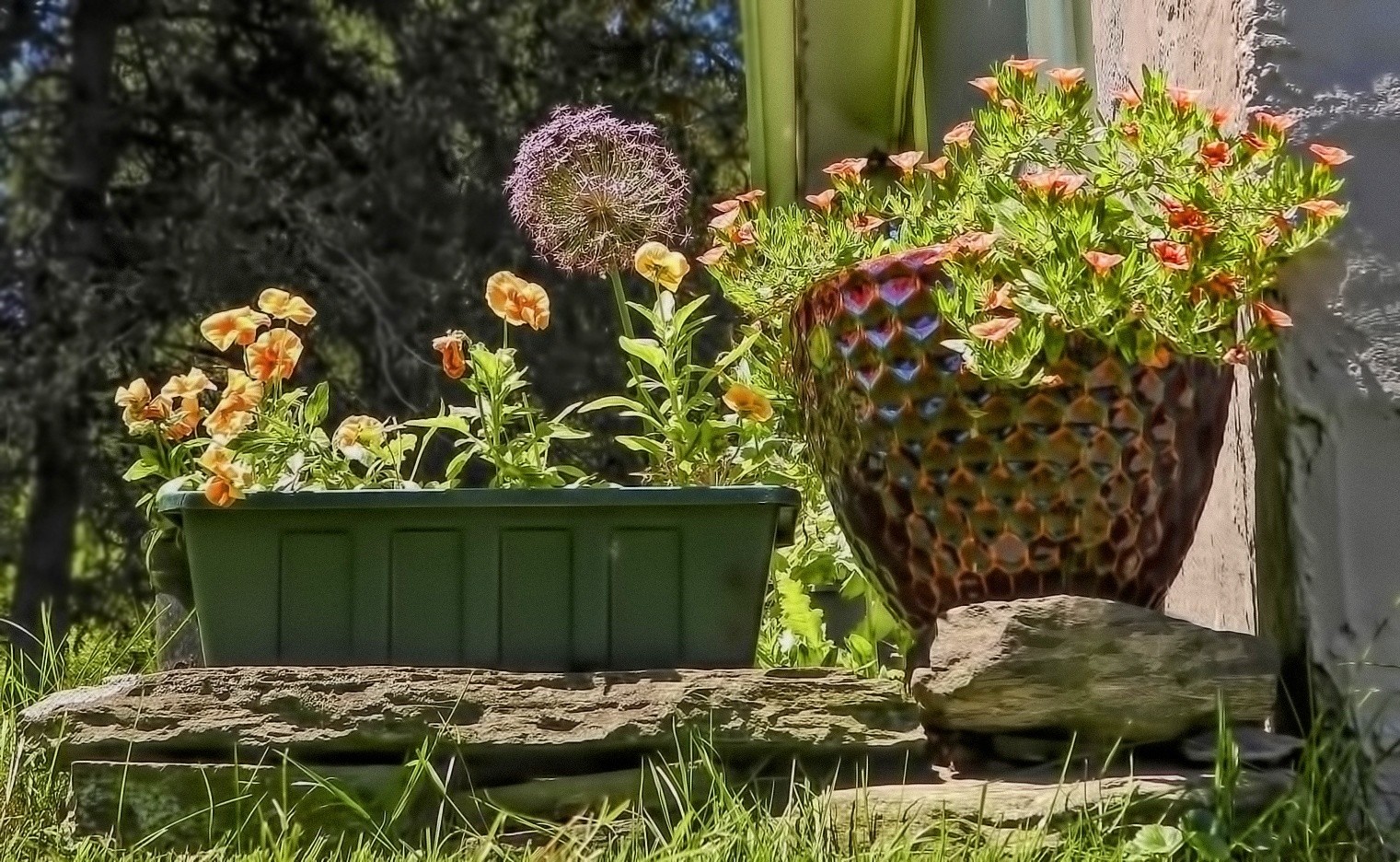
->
[113,109,799,609]
[694,57,1353,389]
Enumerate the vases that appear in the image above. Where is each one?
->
[787,244,1238,639]
[155,484,801,668]
